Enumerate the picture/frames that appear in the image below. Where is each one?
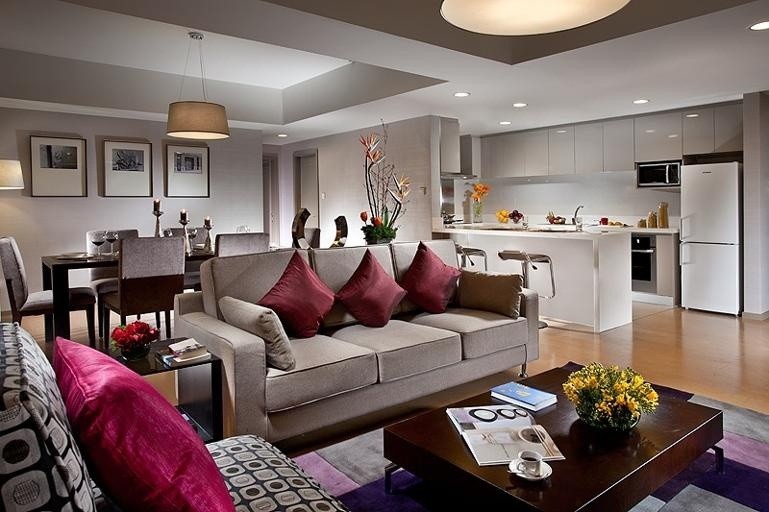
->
[104,140,153,197]
[166,144,210,198]
[30,135,88,197]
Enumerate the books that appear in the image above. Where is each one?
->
[169,337,205,358]
[154,349,211,368]
[446,405,566,466]
[491,382,558,411]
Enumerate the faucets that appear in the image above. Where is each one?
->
[573,204,583,222]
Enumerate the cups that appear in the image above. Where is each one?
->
[516,450,543,477]
[91,230,105,260]
[575,216,583,232]
[105,229,119,253]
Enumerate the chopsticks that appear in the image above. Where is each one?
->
[530,424,556,456]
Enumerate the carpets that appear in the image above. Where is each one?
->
[292,363,769,511]
[632,300,674,321]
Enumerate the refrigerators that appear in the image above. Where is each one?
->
[679,161,743,318]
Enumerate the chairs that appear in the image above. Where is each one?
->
[104,237,185,350]
[86,229,141,338]
[0,237,96,348]
[498,249,555,329]
[213,233,270,257]
[163,228,207,291]
[291,228,320,248]
[454,242,487,272]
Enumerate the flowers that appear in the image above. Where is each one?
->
[470,183,492,217]
[562,362,659,431]
[359,118,410,236]
[110,321,161,351]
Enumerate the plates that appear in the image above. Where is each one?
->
[518,426,547,445]
[509,458,553,480]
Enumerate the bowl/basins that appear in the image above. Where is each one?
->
[510,218,523,224]
[496,218,511,225]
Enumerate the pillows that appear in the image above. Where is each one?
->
[457,267,524,319]
[219,296,296,371]
[52,337,235,512]
[256,249,336,338]
[333,248,408,327]
[399,240,462,313]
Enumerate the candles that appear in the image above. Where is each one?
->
[180,209,188,221]
[154,199,160,214]
[205,216,212,227]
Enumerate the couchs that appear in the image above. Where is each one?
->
[174,239,539,443]
[0,322,349,512]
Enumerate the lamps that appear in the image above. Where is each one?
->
[0,159,24,189]
[166,31,231,140]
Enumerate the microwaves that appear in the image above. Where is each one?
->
[637,162,681,186]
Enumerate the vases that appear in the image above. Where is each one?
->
[576,413,640,453]
[121,344,151,362]
[473,202,482,223]
[363,237,390,244]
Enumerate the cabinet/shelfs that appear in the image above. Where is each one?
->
[683,98,743,155]
[481,130,523,178]
[523,123,575,175]
[634,107,682,162]
[575,114,635,176]
[633,232,680,307]
